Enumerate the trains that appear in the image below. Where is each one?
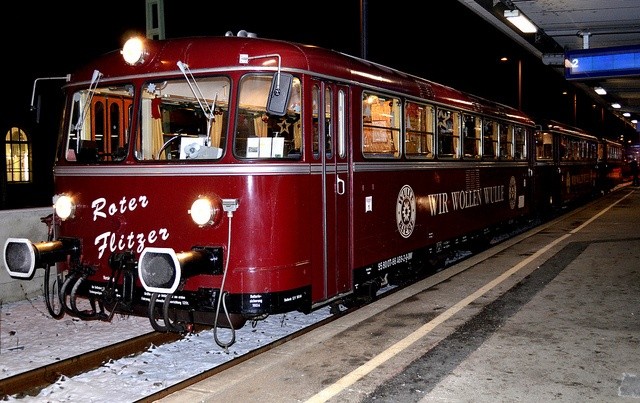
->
[4,29,635,356]
